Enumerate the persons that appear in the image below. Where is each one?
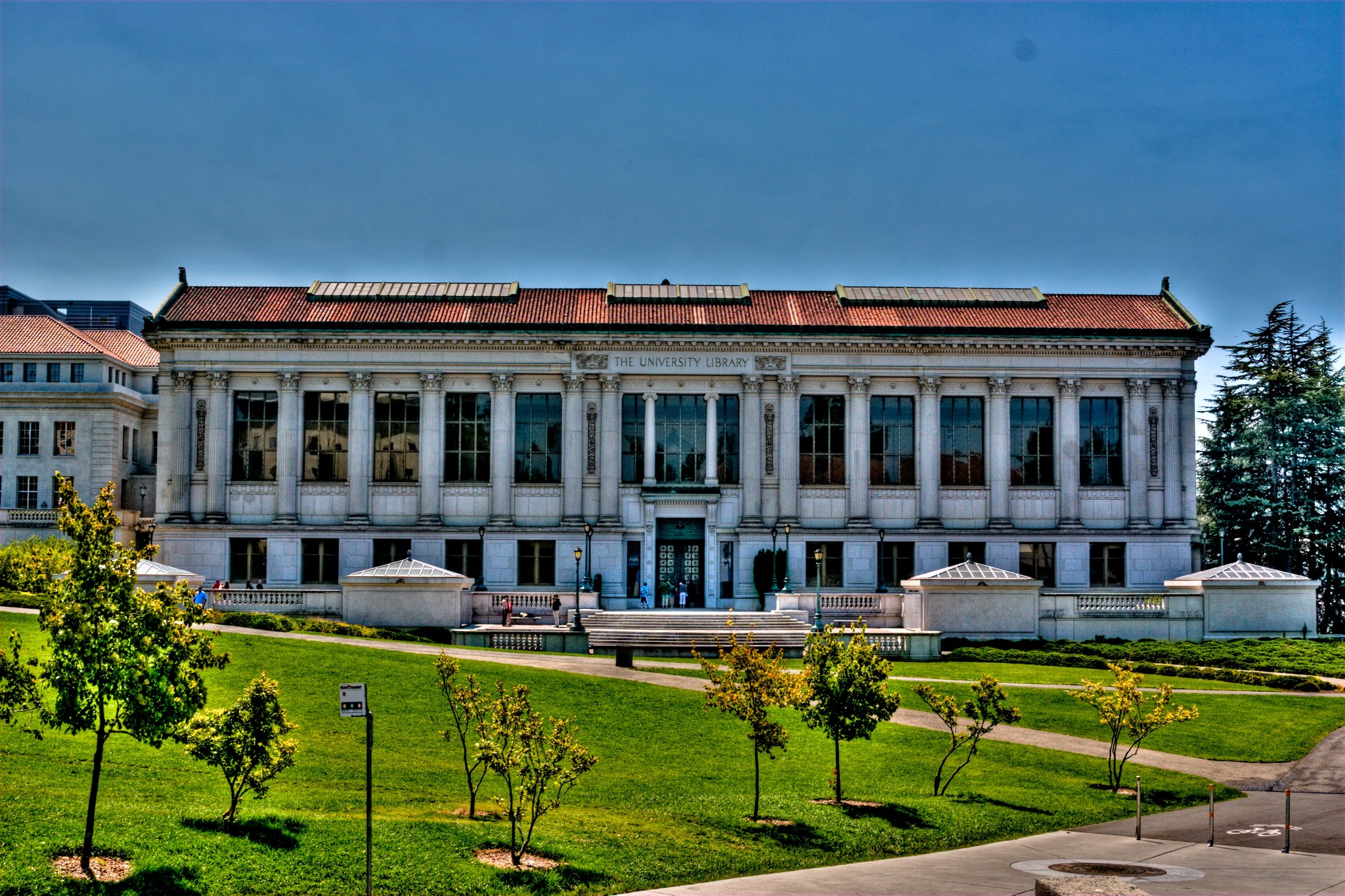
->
[213,579,230,589]
[245,579,264,589]
[659,579,697,608]
[193,586,207,612]
[550,594,562,628]
[501,595,512,627]
[640,582,652,610]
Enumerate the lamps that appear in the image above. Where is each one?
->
[41,501,47,508]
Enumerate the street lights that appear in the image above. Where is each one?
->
[1218,528,1225,566]
[569,546,586,632]
[770,527,780,593]
[588,524,595,592]
[578,521,592,592]
[810,548,827,633]
[1200,529,1208,571]
[781,522,795,594]
[875,527,888,593]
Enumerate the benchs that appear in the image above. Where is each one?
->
[511,617,542,625]
